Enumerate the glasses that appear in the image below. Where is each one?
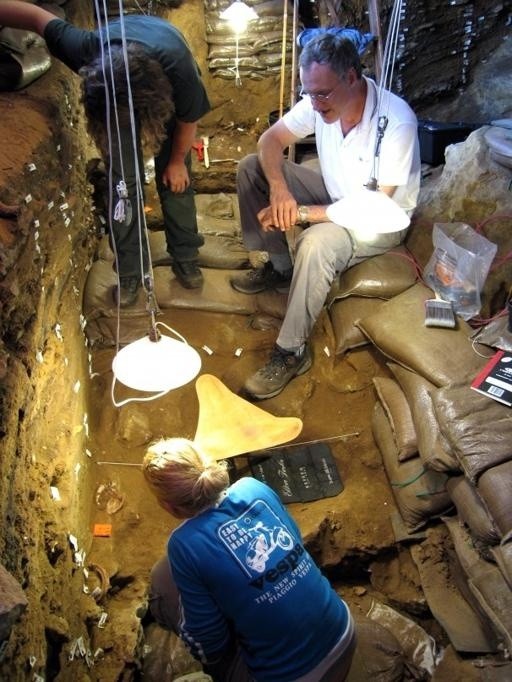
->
[300,84,344,102]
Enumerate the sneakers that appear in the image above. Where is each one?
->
[171,257,205,290]
[115,276,142,307]
[243,347,313,403]
[230,264,298,295]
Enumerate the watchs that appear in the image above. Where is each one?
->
[297,203,309,225]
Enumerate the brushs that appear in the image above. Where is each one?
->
[192,136,210,169]
[425,293,456,328]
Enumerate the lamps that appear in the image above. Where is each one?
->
[110,0,203,392]
[326,1,412,242]
[218,1,260,33]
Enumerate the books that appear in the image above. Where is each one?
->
[470,349,512,405]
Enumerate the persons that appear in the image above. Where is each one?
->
[228,33,422,401]
[141,437,356,682]
[0,1,212,306]
